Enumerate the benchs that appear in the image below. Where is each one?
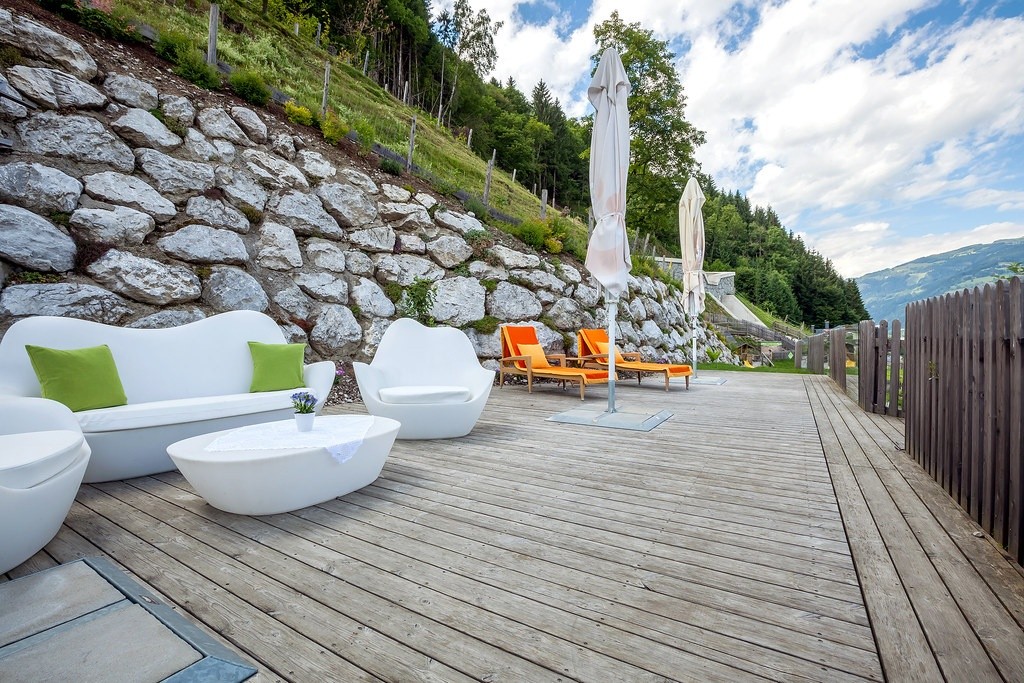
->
[0,309,335,485]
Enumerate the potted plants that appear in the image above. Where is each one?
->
[290,390,316,429]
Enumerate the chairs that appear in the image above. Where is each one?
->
[500,325,617,400]
[0,384,92,575]
[577,329,693,392]
[352,317,495,439]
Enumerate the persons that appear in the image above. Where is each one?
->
[768,350,772,367]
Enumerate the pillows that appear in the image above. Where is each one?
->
[248,339,308,392]
[595,340,625,366]
[517,343,552,369]
[24,342,126,410]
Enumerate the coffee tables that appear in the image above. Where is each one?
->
[167,415,400,516]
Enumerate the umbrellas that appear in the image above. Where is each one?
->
[678,177,706,379]
[588,47,634,410]
